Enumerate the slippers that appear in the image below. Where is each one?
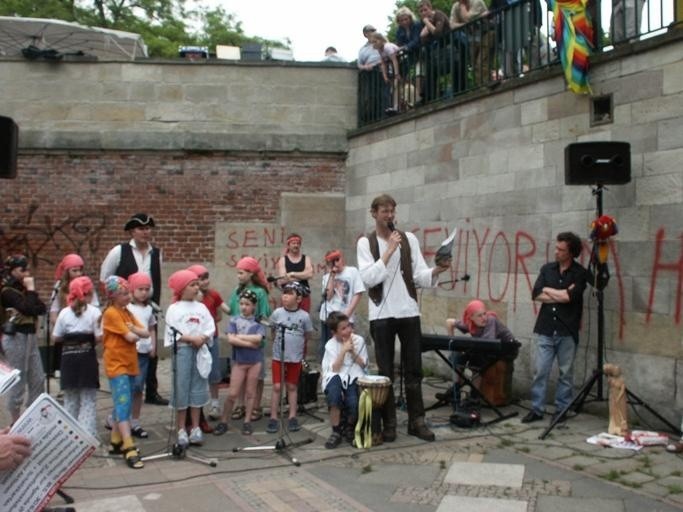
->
[104,418,112,430]
[130,427,148,438]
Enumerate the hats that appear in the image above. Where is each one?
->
[363,24,376,34]
[123,213,156,231]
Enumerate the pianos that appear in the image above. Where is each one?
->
[423,334,519,358]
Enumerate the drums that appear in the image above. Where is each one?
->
[356,374,390,447]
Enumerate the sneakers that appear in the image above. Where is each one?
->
[325,431,342,449]
[265,418,281,433]
[189,427,204,443]
[287,417,299,431]
[177,428,189,446]
[435,387,461,403]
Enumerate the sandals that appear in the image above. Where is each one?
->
[241,422,252,435]
[250,406,264,420]
[231,405,246,420]
[108,440,124,455]
[213,423,228,436]
[122,446,144,468]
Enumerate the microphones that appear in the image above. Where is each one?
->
[146,297,164,313]
[253,312,278,332]
[267,276,286,282]
[438,275,471,287]
[385,220,407,250]
[50,277,63,300]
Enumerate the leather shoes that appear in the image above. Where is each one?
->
[522,410,544,423]
[144,393,169,406]
[551,411,567,423]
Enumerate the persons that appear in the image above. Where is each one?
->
[213,258,274,435]
[100,214,170,468]
[449,0,495,88]
[417,0,450,102]
[163,264,231,447]
[321,47,347,62]
[356,194,453,443]
[48,254,103,444]
[488,1,542,78]
[0,426,32,472]
[608,0,645,50]
[267,233,369,448]
[435,300,521,409]
[356,26,384,128]
[395,6,427,108]
[0,254,45,423]
[521,232,586,424]
[370,33,408,115]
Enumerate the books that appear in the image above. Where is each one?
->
[434,227,457,266]
[0,392,101,512]
[0,362,22,399]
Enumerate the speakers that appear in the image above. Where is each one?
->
[563,140,633,186]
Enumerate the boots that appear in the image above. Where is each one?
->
[407,413,436,442]
[382,412,398,442]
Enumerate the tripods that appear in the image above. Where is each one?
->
[537,261,683,440]
[265,337,326,424]
[138,332,219,467]
[232,329,315,467]
